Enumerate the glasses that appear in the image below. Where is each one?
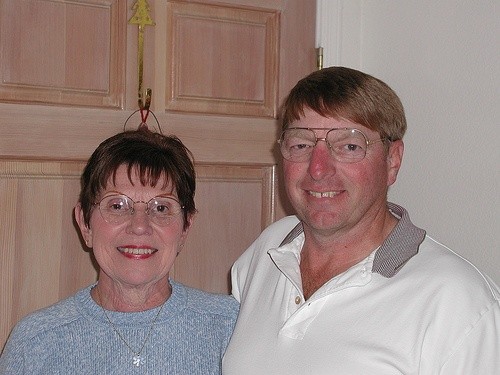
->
[91,195,185,226]
[277,128,388,163]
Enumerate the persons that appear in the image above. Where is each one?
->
[0,125,241,375]
[222,66,500,375]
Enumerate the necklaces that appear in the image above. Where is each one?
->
[95,283,171,368]
